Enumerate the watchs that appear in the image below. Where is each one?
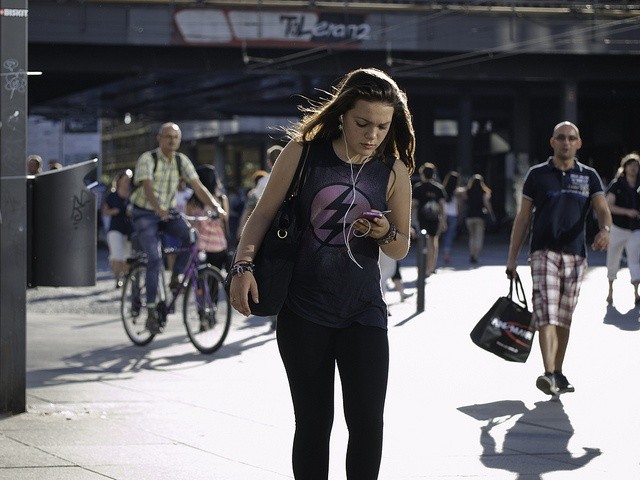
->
[597,224,611,232]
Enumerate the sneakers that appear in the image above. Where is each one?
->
[536,373,561,395]
[555,375,575,393]
[146,317,161,331]
[168,282,188,290]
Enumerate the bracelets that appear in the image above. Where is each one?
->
[375,223,396,245]
[226,261,257,279]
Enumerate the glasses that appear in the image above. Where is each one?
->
[554,134,578,141]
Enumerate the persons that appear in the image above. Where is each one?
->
[104,171,134,289]
[228,68,415,480]
[441,170,463,263]
[187,162,233,331]
[465,173,492,260]
[131,124,225,332]
[410,162,447,280]
[605,154,640,307]
[506,121,613,397]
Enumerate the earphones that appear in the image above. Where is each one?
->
[338,114,351,134]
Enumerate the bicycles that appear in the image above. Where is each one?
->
[121,210,233,356]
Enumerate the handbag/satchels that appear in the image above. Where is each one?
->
[470,268,540,362]
[223,139,316,315]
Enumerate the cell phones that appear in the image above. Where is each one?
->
[357,208,389,236]
